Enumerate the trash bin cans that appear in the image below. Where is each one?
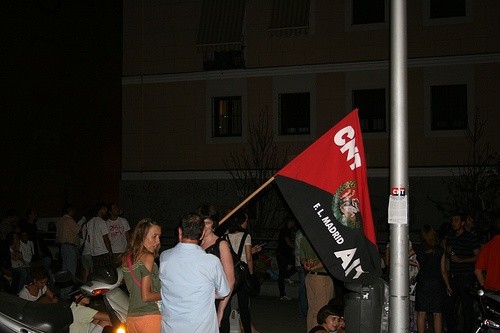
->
[343,278,385,333]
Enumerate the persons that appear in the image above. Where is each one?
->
[159,211,235,333]
[18,266,58,303]
[122,217,162,333]
[68,289,113,333]
[40,204,129,287]
[386,212,500,333]
[0,208,39,284]
[278,211,345,333]
[219,210,261,333]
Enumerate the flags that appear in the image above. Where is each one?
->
[274,108,382,292]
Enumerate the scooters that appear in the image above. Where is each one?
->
[0,265,131,333]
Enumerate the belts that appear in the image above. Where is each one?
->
[308,271,329,276]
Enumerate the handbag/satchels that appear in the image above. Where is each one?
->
[229,309,241,332]
[238,274,260,297]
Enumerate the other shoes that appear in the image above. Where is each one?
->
[280,296,292,300]
[285,278,293,283]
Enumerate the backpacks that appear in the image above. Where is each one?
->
[223,232,249,296]
